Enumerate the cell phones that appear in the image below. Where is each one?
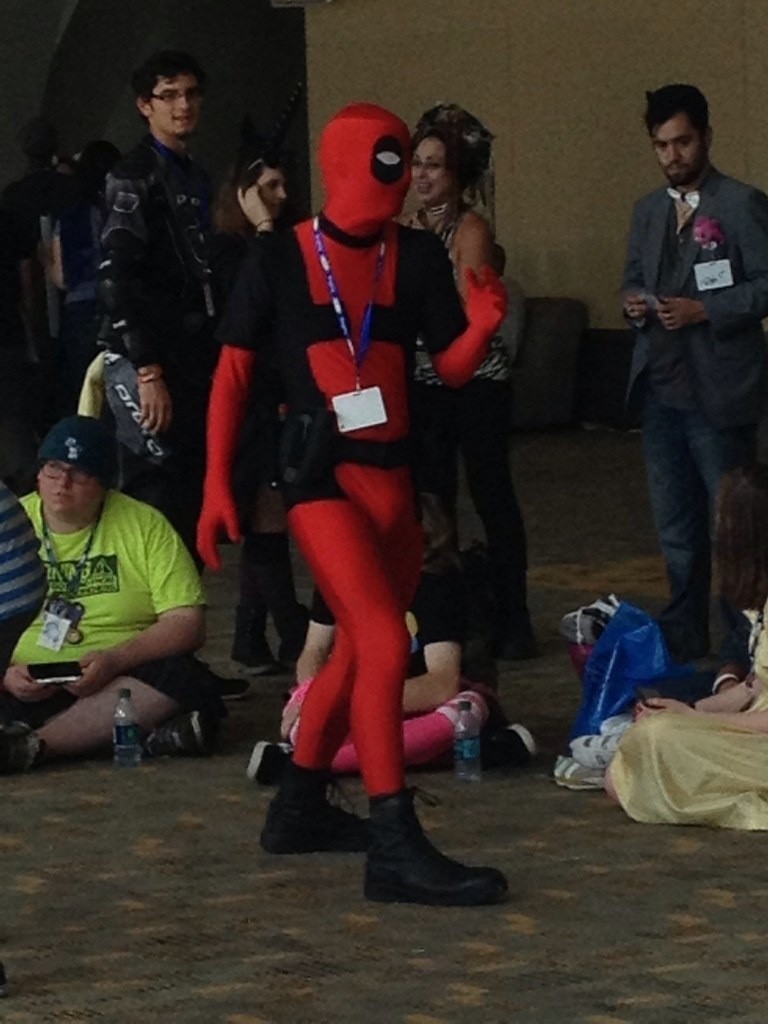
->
[35,675,83,684]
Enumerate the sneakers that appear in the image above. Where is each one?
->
[143,710,210,758]
[550,757,604,792]
[0,720,41,775]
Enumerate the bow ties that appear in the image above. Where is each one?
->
[666,187,701,209]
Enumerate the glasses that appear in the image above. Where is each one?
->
[410,157,448,176]
[247,151,279,173]
[39,457,92,486]
[151,87,203,103]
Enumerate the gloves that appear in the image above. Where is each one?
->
[464,264,509,336]
[194,481,240,572]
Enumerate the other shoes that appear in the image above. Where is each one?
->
[246,740,294,786]
[200,665,252,702]
[504,722,537,763]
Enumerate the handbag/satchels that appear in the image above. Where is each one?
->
[560,591,676,749]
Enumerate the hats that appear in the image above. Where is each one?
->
[37,414,119,480]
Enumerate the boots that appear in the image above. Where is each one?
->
[270,601,310,668]
[229,605,281,676]
[258,755,364,856]
[362,784,509,907]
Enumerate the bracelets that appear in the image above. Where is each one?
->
[709,672,738,694]
[135,374,161,383]
[255,218,272,228]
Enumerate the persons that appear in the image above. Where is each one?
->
[98,49,218,577]
[193,104,510,903]
[621,81,768,666]
[245,495,536,784]
[391,103,532,658]
[605,461,768,830]
[0,120,107,497]
[213,147,307,674]
[0,413,216,772]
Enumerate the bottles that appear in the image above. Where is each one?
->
[112,689,141,766]
[452,701,480,781]
